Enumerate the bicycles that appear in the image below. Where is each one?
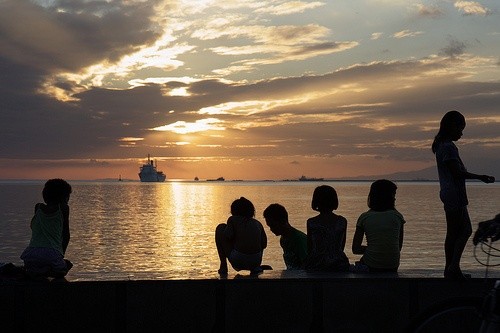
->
[406,221,500,333]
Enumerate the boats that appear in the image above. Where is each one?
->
[137,153,167,182]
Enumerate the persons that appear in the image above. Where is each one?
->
[432,111,496,283]
[20,178,73,282]
[352,179,406,275]
[306,185,350,272]
[264,204,307,271]
[215,196,267,277]
